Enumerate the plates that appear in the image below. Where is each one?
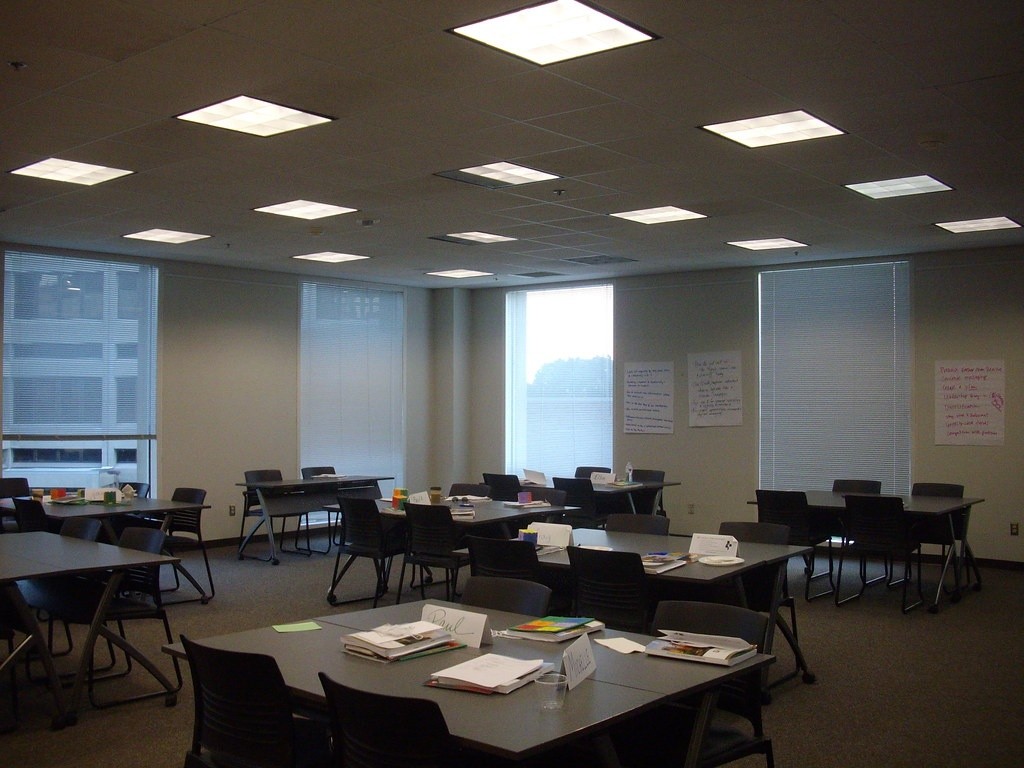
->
[698,556,745,567]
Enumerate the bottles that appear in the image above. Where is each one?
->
[625,461,633,482]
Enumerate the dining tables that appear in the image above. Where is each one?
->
[238,470,312,562]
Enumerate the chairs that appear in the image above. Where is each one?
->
[809,479,888,585]
[331,497,778,768]
[627,468,666,517]
[0,477,216,709]
[575,467,611,479]
[295,466,350,555]
[888,482,970,595]
[179,633,333,768]
[449,472,925,690]
[317,671,468,768]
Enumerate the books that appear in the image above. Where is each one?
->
[640,551,690,574]
[438,663,556,693]
[450,509,475,519]
[505,615,605,642]
[645,629,757,667]
[606,480,643,489]
[340,619,467,664]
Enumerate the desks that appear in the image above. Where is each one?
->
[322,497,583,597]
[479,481,680,530]
[237,475,394,565]
[313,597,778,768]
[747,490,985,613]
[508,528,816,705]
[163,619,668,768]
[0,494,211,732]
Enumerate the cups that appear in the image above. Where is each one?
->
[104,491,116,504]
[518,491,532,503]
[534,674,569,710]
[77,489,85,498]
[123,492,134,505]
[31,489,44,505]
[430,487,442,505]
